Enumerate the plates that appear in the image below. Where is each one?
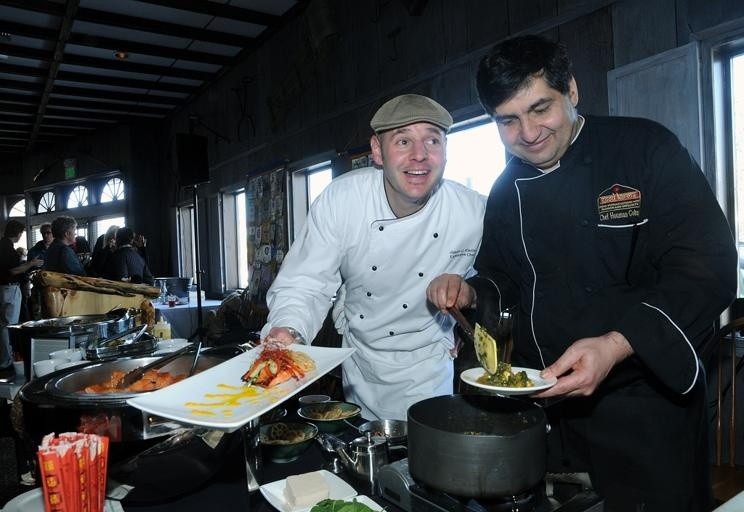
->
[123,340,359,434]
[2,487,128,512]
[258,468,387,512]
[457,365,560,401]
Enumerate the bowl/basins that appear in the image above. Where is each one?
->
[43,354,226,401]
[360,420,408,445]
[77,333,159,360]
[12,347,92,376]
[258,393,363,465]
[153,276,194,298]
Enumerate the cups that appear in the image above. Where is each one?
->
[167,294,177,307]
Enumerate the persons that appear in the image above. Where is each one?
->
[258,88,486,438]
[426,31,738,510]
[0,214,157,373]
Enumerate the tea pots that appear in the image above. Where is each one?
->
[337,430,407,496]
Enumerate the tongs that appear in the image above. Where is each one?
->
[116,340,203,389]
[94,323,149,345]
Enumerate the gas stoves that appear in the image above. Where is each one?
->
[377,456,607,512]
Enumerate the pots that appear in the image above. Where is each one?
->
[407,394,548,497]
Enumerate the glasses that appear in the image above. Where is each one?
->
[42,231,51,235]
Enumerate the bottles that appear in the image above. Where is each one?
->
[150,279,168,304]
[154,315,172,340]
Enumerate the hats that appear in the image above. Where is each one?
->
[369,93,453,133]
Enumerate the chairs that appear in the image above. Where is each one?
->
[208,286,251,345]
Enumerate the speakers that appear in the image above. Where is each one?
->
[176,136,210,192]
